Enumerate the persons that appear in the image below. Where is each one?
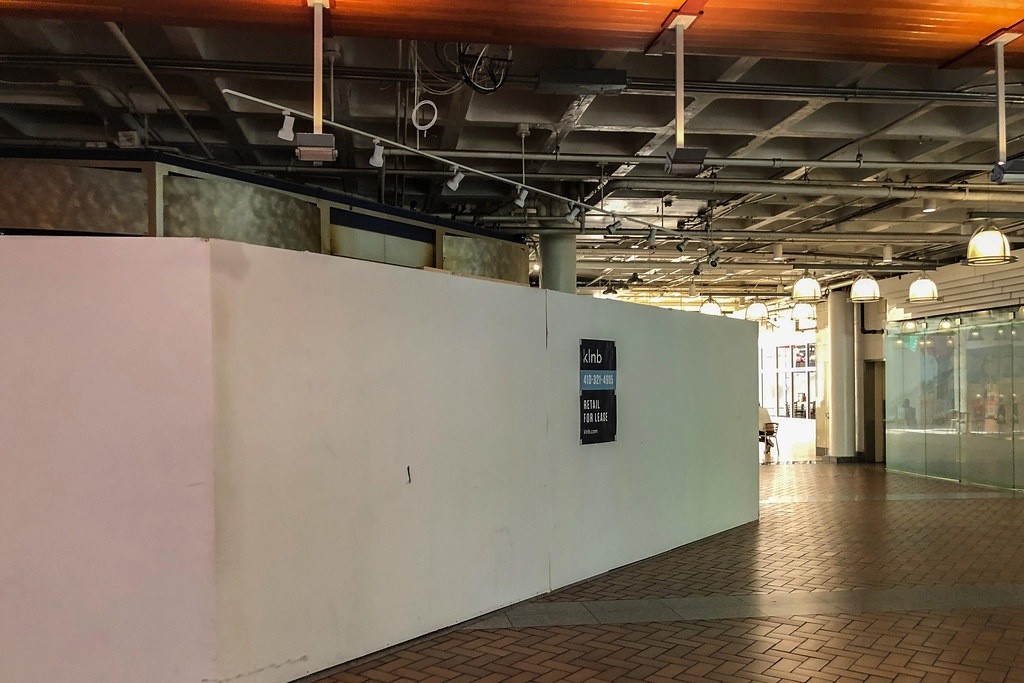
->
[904,399,915,425]
[801,393,806,413]
[998,394,1006,432]
[1013,393,1019,432]
[974,395,985,432]
[759,402,775,462]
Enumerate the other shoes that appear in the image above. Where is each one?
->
[766,439,774,447]
[764,445,770,454]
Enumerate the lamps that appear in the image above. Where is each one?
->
[790,268,828,303]
[698,296,722,316]
[369,139,385,168]
[603,272,616,294]
[446,165,465,191]
[745,296,771,321]
[565,202,581,224]
[960,217,1018,266]
[923,198,936,213]
[676,237,688,253]
[790,303,816,319]
[905,270,943,304]
[607,214,622,234]
[846,272,885,304]
[709,256,720,267]
[773,245,781,260]
[514,184,528,208]
[627,272,644,284]
[646,225,657,243]
[883,247,892,263]
[693,263,703,275]
[277,111,295,142]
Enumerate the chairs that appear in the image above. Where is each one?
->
[758,422,780,457]
